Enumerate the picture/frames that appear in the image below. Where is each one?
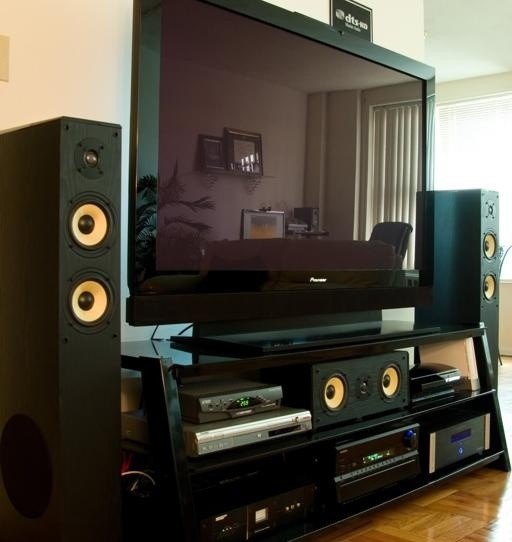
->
[223,126,263,174]
[199,134,224,173]
[241,208,285,240]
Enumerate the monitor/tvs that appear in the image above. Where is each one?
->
[125,0,437,354]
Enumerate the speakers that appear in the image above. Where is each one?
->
[414,189,501,396]
[281,350,410,432]
[0,115,122,541]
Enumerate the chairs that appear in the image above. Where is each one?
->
[368,221,413,261]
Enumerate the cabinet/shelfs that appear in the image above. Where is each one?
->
[121,320,511,541]
